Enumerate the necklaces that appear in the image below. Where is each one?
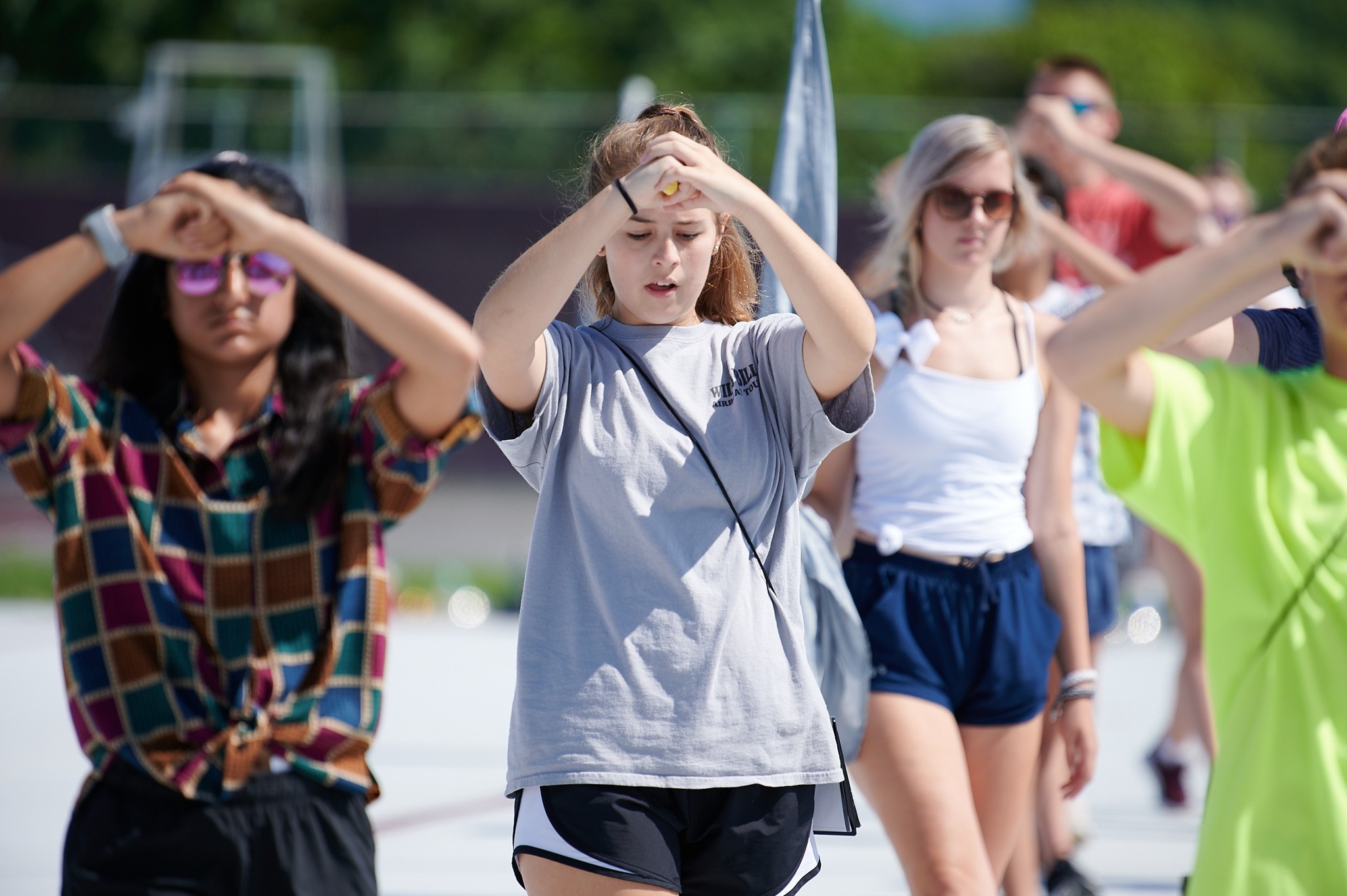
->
[918,277,994,324]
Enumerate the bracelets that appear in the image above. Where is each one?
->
[80,203,134,271]
[615,176,638,216]
[1061,669,1097,689]
[1053,687,1095,715]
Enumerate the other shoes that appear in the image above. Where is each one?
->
[1146,748,1185,805]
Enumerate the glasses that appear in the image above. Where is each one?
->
[923,183,1019,225]
[170,247,292,298]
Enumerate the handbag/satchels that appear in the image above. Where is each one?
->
[809,717,860,841]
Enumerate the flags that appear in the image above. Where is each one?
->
[755,0,838,320]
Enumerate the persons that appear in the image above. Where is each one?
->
[0,150,479,896]
[799,55,1347,895]
[471,102,877,895]
